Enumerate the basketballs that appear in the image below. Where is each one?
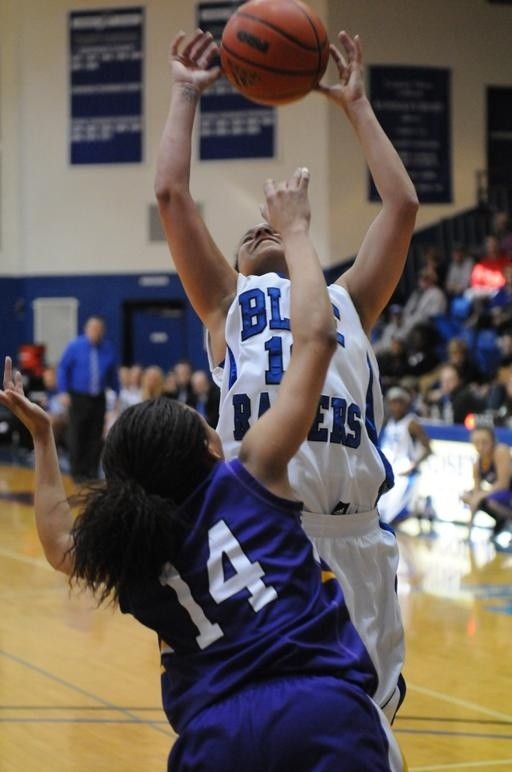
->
[221,0,330,106]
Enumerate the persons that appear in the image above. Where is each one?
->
[55,314,122,487]
[153,22,424,772]
[372,383,439,528]
[365,205,510,435]
[0,344,220,456]
[456,424,512,549]
[0,162,402,770]
